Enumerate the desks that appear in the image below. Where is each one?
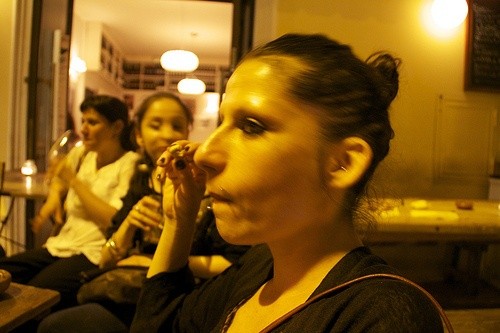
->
[0,282,61,333]
[359,197,500,266]
[0,167,51,250]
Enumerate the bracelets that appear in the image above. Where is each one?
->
[106,238,123,261]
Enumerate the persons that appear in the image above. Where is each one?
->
[132,31,448,332]
[36,90,254,332]
[1,92,138,308]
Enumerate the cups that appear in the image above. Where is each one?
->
[143,195,165,243]
